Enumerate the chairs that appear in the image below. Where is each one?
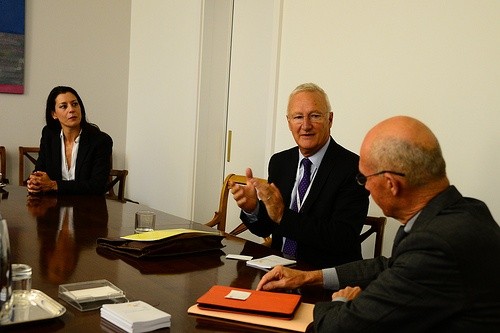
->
[203,174,273,247]
[105,170,128,203]
[360,217,387,258]
[18,146,40,187]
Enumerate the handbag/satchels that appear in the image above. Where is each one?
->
[96,228,226,260]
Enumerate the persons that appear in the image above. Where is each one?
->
[223,82,371,270]
[256,116,500,333]
[26,197,108,283]
[27,85,113,196]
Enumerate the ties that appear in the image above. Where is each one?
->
[282,158,313,257]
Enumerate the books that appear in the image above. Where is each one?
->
[197,285,303,320]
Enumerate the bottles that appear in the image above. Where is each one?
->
[0,216,14,322]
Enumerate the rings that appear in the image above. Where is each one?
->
[267,196,272,200]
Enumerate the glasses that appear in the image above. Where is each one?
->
[356,170,405,185]
[287,111,327,122]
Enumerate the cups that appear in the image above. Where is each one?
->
[133,212,157,235]
[7,264,33,307]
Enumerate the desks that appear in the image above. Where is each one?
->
[0,186,337,333]
